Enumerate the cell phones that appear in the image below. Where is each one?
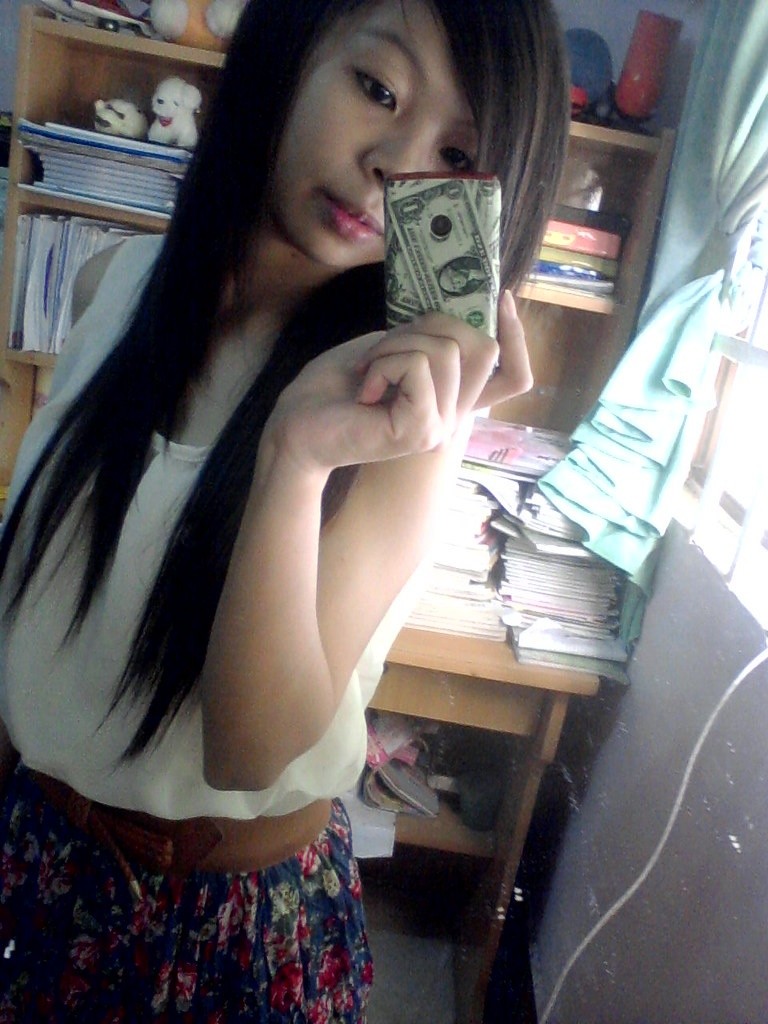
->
[384,170,502,379]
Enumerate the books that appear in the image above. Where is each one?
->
[17,117,189,221]
[8,211,147,354]
[516,218,620,298]
[401,415,635,687]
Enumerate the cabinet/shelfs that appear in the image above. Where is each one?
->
[0,4,677,1023]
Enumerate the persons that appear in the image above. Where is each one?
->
[0,1,570,1024]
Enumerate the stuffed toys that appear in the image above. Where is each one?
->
[147,75,202,147]
[149,1,246,52]
[93,98,148,140]
